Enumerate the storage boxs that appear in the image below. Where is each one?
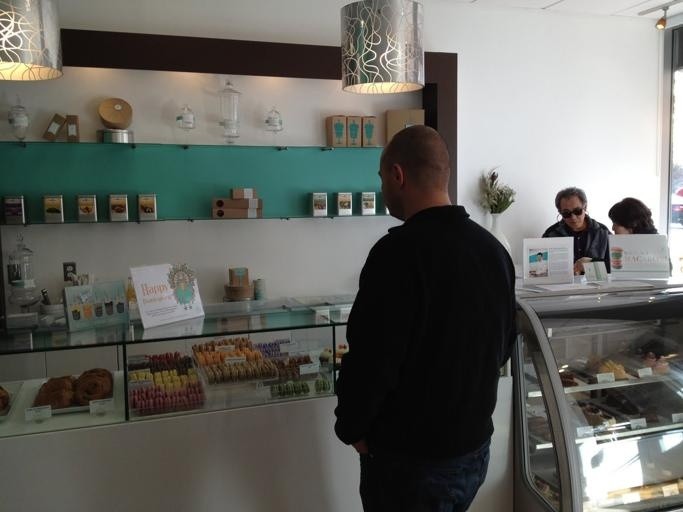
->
[211,188,262,219]
[327,107,425,147]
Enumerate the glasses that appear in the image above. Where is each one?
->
[558,205,585,219]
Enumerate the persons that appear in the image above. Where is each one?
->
[541,186,614,276]
[333,123,519,512]
[530,252,547,276]
[573,198,675,275]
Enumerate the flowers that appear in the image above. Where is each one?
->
[479,166,516,213]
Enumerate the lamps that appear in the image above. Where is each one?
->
[339,0,426,94]
[1,0,69,82]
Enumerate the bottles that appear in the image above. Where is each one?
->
[8,94,31,141]
[253,278,265,300]
[6,233,42,309]
[125,278,135,300]
[216,80,241,143]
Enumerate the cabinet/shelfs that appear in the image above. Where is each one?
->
[514,285,683,511]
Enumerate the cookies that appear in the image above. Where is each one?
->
[128,337,331,416]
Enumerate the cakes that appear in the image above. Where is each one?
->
[318,340,348,366]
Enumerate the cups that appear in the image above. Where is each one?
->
[39,297,66,318]
[70,296,125,321]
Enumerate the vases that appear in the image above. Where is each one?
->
[490,214,511,257]
